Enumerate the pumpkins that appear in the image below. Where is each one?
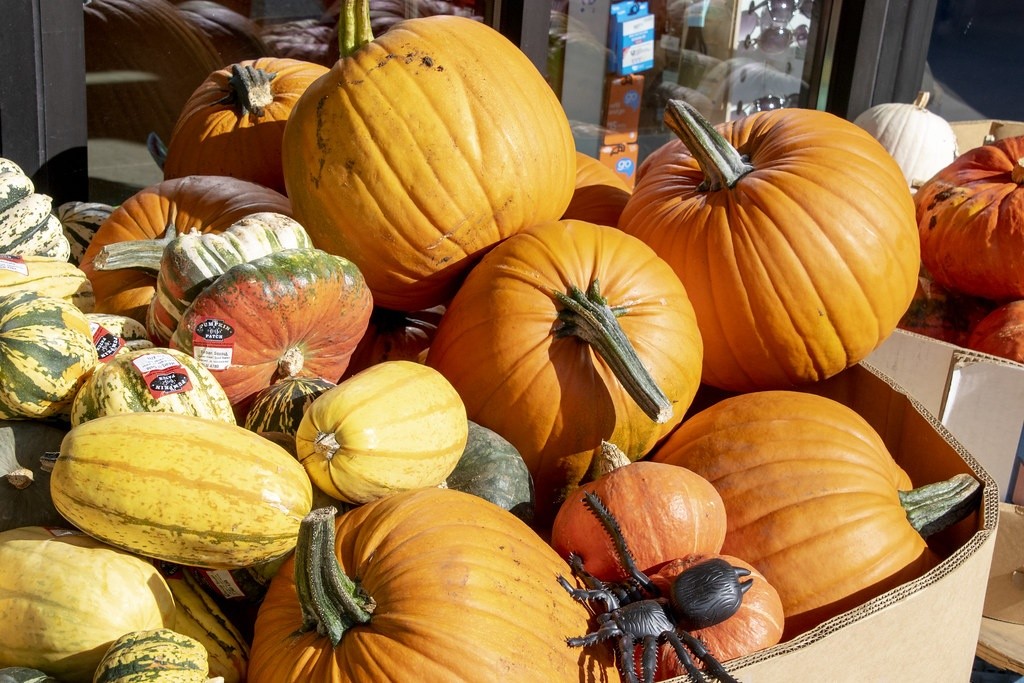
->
[0,0,1024,683]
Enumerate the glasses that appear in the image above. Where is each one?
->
[745,25,809,53]
[736,93,805,113]
[748,0,812,22]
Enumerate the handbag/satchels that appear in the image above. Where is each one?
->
[660,34,680,52]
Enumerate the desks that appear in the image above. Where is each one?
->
[971,502,1024,676]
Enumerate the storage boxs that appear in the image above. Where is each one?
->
[864,120,1024,507]
[622,359,998,683]
